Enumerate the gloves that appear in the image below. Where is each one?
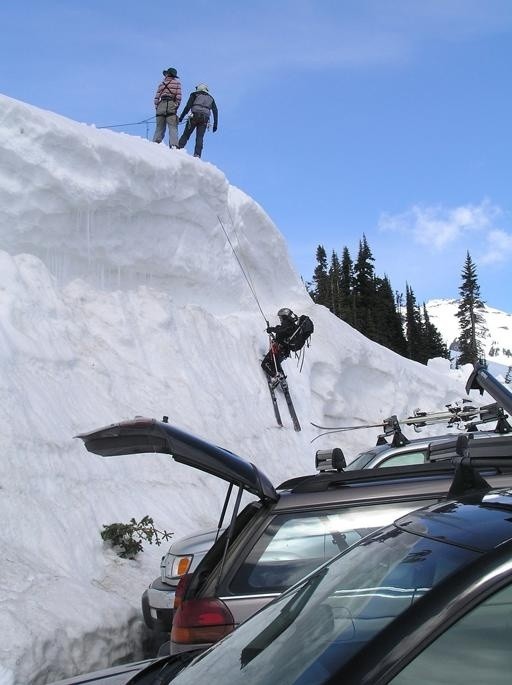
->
[267,327,274,334]
[213,127,217,132]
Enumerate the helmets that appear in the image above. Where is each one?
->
[277,308,291,319]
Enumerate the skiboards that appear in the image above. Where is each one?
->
[308,399,508,444]
[261,357,301,432]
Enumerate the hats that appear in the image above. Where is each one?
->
[163,68,179,79]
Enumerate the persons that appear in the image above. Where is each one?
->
[261,308,312,392]
[179,83,218,157]
[151,67,183,149]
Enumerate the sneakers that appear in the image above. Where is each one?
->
[268,370,288,389]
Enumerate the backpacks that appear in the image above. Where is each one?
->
[276,315,314,352]
